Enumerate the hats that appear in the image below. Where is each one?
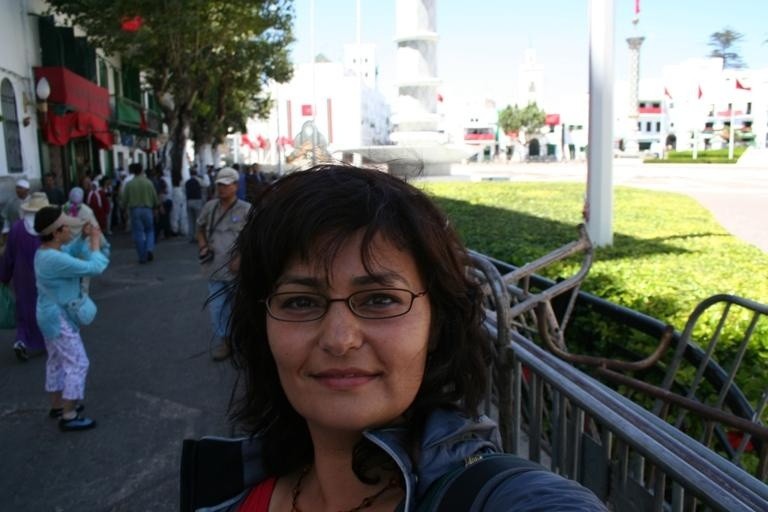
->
[20,192,58,211]
[40,211,81,236]
[216,166,240,186]
[15,179,31,188]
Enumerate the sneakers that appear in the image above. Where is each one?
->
[48,403,85,418]
[59,414,96,431]
[210,337,228,361]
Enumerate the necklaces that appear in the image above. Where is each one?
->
[292,464,394,511]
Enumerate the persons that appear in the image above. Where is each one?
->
[38,174,66,205]
[119,163,171,261]
[146,165,173,197]
[64,189,101,295]
[120,164,135,200]
[193,165,254,360]
[183,164,209,242]
[179,165,610,512]
[0,193,49,360]
[206,164,215,188]
[232,163,246,199]
[81,167,124,235]
[242,165,264,188]
[34,206,107,429]
[2,180,31,227]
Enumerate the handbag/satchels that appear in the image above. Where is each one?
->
[36,281,98,326]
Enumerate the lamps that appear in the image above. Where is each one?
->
[22,76,51,125]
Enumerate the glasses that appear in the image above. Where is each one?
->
[264,287,428,322]
